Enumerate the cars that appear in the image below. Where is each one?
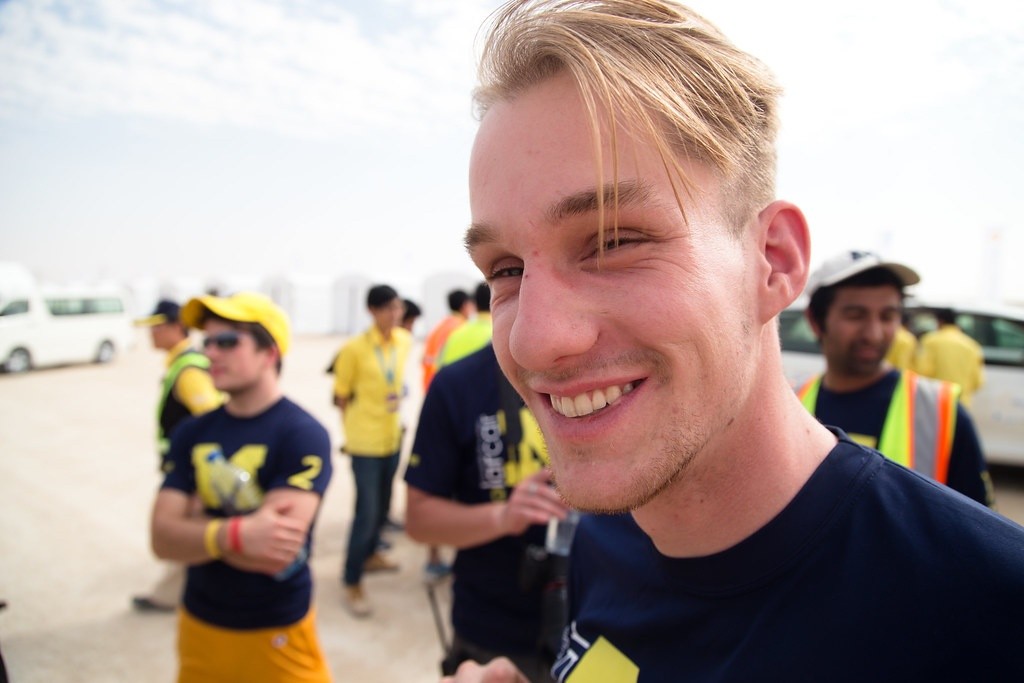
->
[779,291,1024,472]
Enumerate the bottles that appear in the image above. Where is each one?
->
[205,451,309,583]
[542,505,582,556]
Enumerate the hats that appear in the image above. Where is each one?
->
[802,252,921,296]
[127,302,184,326]
[179,293,289,362]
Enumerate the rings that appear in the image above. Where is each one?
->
[528,482,538,492]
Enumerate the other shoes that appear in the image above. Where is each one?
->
[133,594,181,611]
[348,583,369,616]
[381,519,404,531]
[366,553,399,569]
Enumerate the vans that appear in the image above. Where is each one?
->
[0,289,139,377]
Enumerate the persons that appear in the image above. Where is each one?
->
[326,284,422,613]
[131,300,229,612]
[884,313,917,369]
[912,307,985,406]
[149,290,332,682]
[421,290,472,390]
[439,0,1024,683]
[793,250,995,513]
[435,283,494,370]
[404,340,568,683]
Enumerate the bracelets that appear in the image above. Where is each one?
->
[202,516,222,560]
[230,518,242,554]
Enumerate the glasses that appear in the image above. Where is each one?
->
[202,333,239,349]
